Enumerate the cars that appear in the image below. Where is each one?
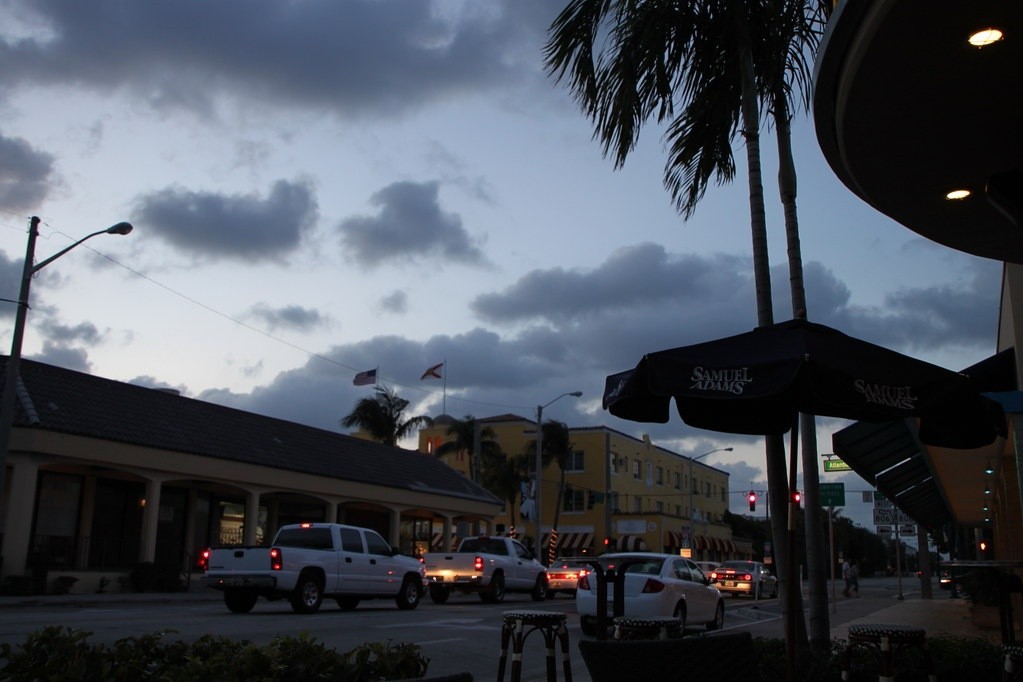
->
[710,560,778,601]
[547,556,598,595]
[677,560,722,581]
[576,553,726,640]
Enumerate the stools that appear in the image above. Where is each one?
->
[611,614,683,641]
[840,624,938,682]
[495,610,573,682]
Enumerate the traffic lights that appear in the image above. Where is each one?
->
[749,492,756,512]
[794,491,801,509]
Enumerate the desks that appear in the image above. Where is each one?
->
[937,559,1023,654]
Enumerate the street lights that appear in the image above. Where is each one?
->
[687,447,734,559]
[1,216,132,484]
[535,390,584,562]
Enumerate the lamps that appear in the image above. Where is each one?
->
[983,456,994,474]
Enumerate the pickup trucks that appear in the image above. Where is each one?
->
[418,534,549,606]
[199,522,429,616]
